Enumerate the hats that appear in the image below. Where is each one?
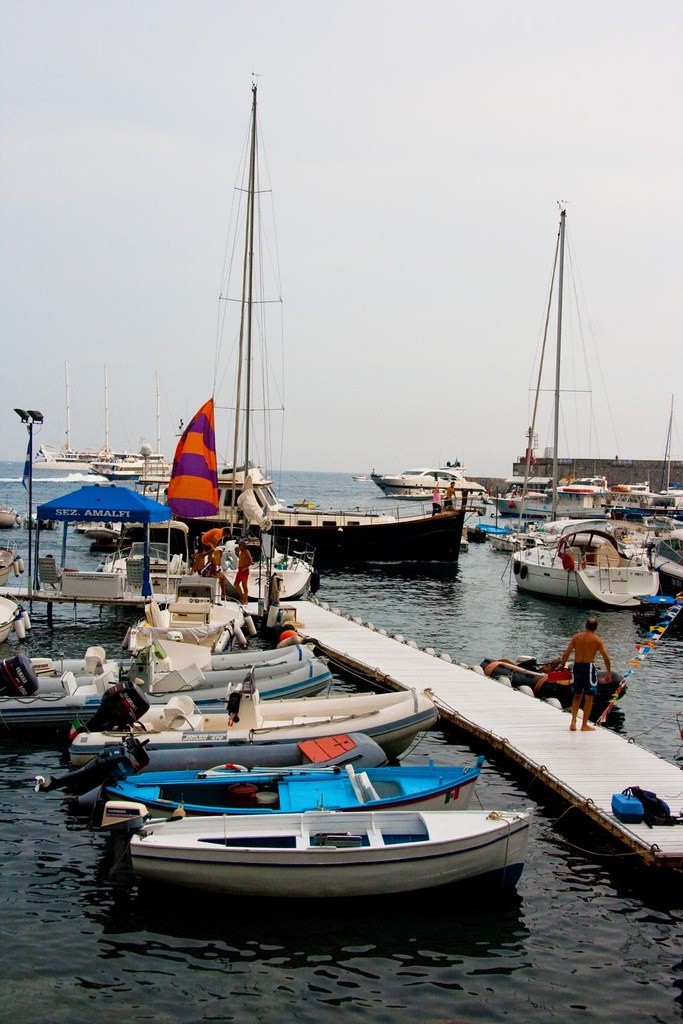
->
[198,546,205,554]
[238,539,246,546]
[223,528,233,539]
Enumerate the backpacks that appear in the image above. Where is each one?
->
[629,786,677,829]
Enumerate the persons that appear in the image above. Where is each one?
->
[444,482,456,511]
[234,540,252,606]
[46,555,78,576]
[432,482,442,517]
[557,618,611,730]
[188,526,231,600]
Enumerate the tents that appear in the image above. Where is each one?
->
[32,486,171,597]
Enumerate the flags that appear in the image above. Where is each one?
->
[20,433,32,492]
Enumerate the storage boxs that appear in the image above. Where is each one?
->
[325,835,362,847]
[313,832,352,845]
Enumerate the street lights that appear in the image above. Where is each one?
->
[14,407,44,587]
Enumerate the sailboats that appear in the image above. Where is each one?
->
[91,75,486,568]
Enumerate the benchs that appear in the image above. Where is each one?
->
[170,613,206,628]
[296,831,310,850]
[367,828,386,845]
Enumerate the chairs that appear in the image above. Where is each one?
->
[60,671,97,695]
[38,558,63,597]
[124,560,146,599]
[165,696,204,732]
[84,646,106,674]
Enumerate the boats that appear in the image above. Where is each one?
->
[372,207,682,635]
[0,355,334,740]
[481,652,626,711]
[100,798,535,904]
[64,662,440,774]
[38,735,485,830]
[353,474,368,483]
